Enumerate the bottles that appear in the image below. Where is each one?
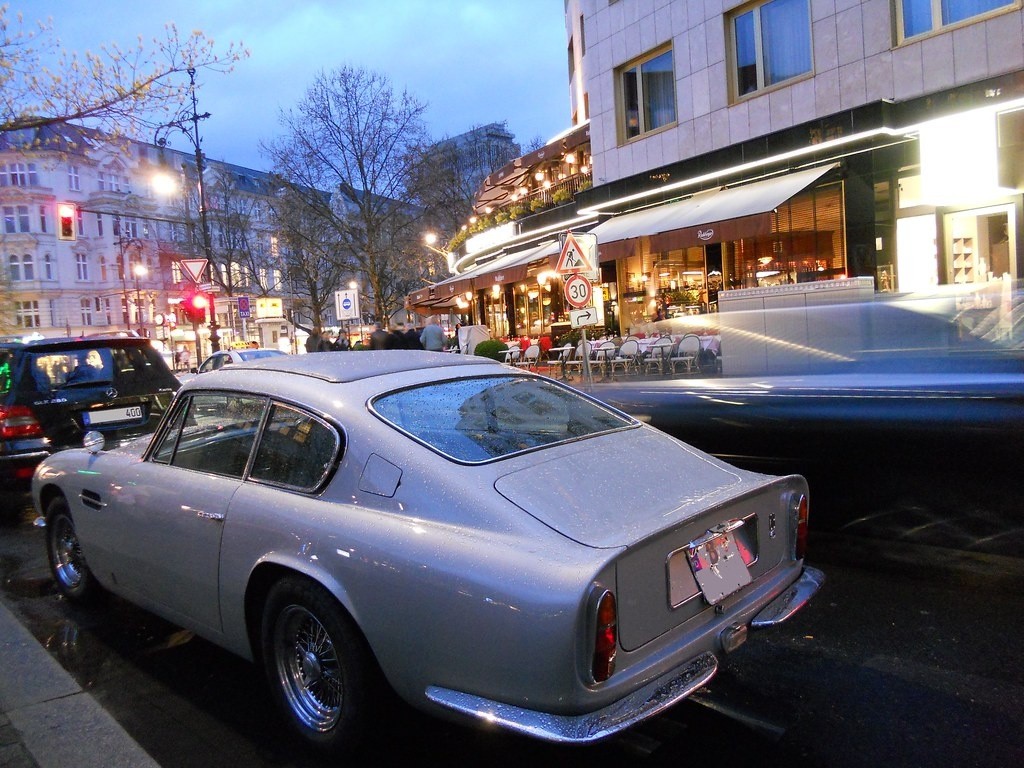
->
[742,253,830,279]
[558,313,568,322]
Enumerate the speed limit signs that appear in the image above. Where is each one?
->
[566,274,592,309]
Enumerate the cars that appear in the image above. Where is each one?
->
[32,349,825,763]
[347,324,375,349]
[173,341,289,415]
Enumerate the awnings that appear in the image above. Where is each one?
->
[404,298,469,315]
[587,162,840,262]
[434,243,554,299]
[473,241,561,290]
[411,255,504,306]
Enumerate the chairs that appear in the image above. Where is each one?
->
[505,333,700,376]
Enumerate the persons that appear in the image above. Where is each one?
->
[175,345,190,373]
[654,307,672,337]
[420,316,447,351]
[370,322,425,350]
[306,327,353,352]
[53,353,97,382]
[455,323,462,335]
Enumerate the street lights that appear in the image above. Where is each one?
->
[155,63,225,371]
[113,215,147,329]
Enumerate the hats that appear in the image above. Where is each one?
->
[340,328,347,333]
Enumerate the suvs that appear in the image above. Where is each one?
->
[0,326,198,493]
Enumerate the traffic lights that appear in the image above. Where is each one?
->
[180,295,205,323]
[55,202,75,242]
